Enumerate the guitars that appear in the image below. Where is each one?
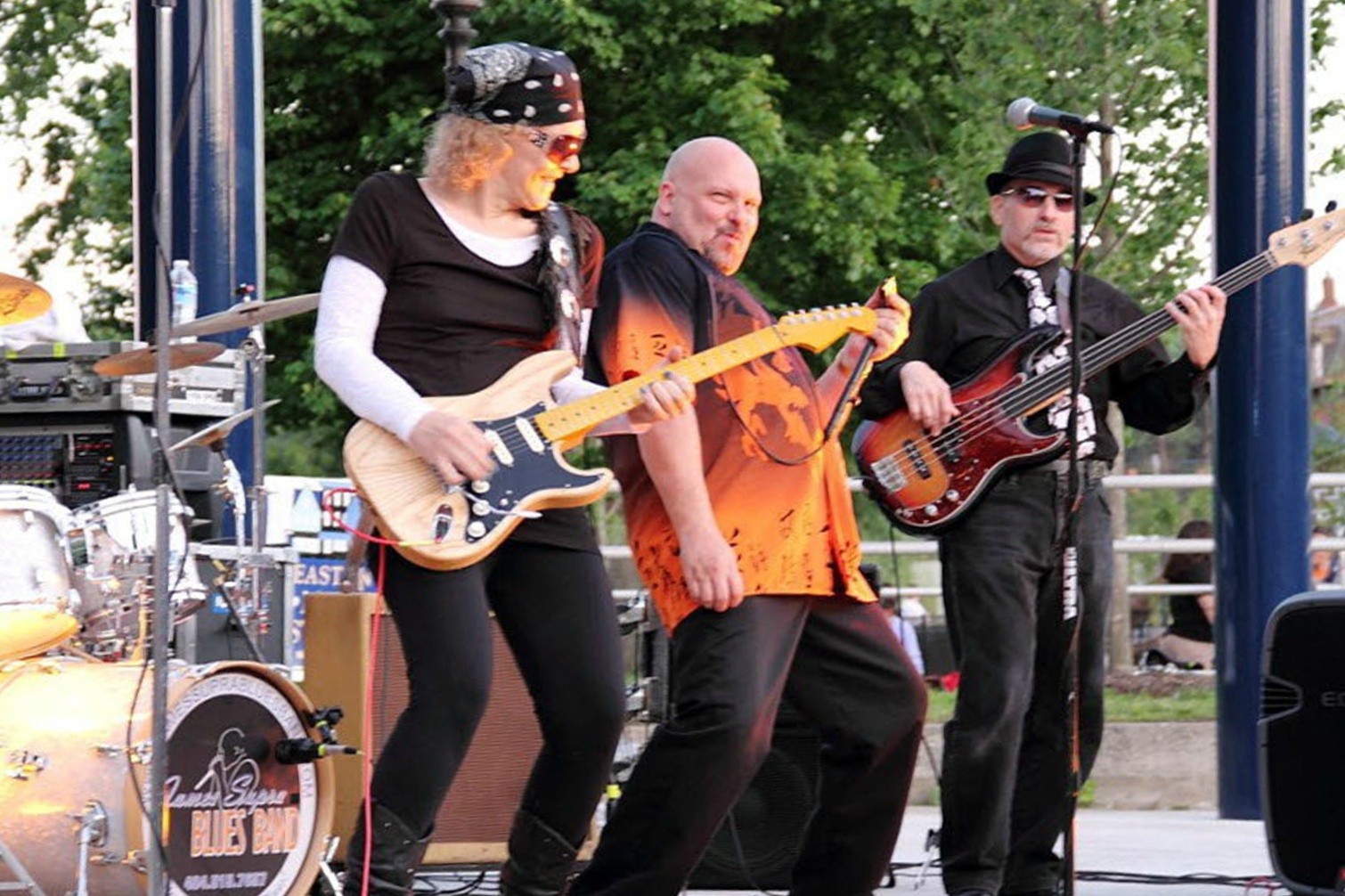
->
[850,199,1345,537]
[340,301,879,572]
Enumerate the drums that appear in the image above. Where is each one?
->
[1,661,339,896]
[0,482,82,659]
[68,486,208,645]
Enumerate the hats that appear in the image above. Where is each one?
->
[985,131,1098,207]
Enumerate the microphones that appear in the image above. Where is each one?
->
[276,738,358,765]
[1007,98,1114,135]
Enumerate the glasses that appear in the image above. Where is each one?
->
[519,125,585,165]
[998,184,1075,212]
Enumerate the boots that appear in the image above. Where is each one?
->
[502,806,577,896]
[344,798,435,896]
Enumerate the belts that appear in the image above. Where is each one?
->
[1040,459,1107,476]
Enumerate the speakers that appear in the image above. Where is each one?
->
[1257,589,1345,896]
[304,592,595,864]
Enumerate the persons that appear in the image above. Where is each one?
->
[860,133,1227,896]
[1138,520,1218,667]
[566,135,929,895]
[1311,526,1345,591]
[315,43,698,896]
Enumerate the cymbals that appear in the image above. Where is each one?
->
[171,292,321,338]
[0,269,53,327]
[165,396,284,456]
[92,341,226,379]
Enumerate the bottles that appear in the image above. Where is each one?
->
[167,258,197,347]
